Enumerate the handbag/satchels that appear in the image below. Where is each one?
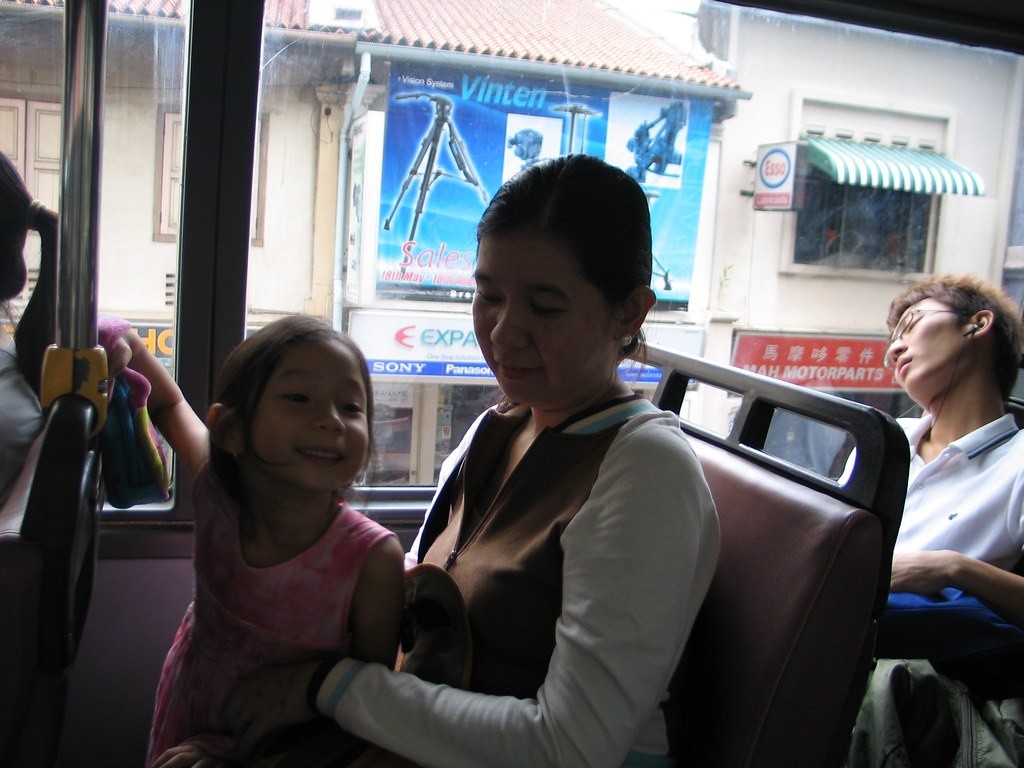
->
[845,587,1023,768]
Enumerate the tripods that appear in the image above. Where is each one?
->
[384,92,492,281]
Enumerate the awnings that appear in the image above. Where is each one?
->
[804,135,986,197]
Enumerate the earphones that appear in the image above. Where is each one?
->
[963,324,983,338]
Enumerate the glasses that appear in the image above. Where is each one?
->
[883,309,958,369]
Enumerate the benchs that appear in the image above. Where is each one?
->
[615,338,910,768]
[0,396,100,768]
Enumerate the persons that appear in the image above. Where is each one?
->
[0,145,721,768]
[839,275,1024,617]
